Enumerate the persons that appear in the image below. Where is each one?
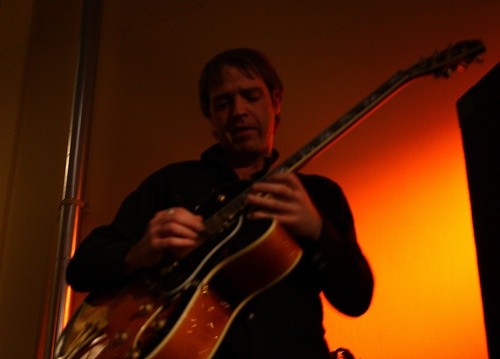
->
[65,46,374,359]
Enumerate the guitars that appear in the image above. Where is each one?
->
[53,39,487,359]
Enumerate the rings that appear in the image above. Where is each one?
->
[168,207,174,215]
